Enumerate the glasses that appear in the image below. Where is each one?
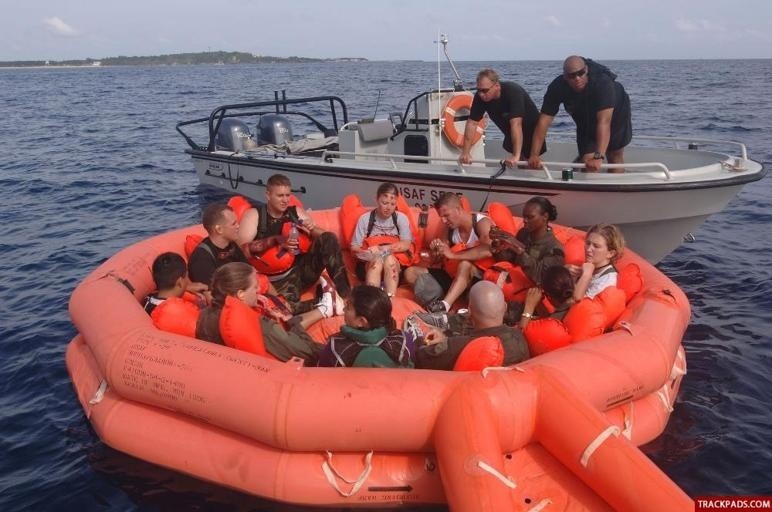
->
[478,81,497,93]
[566,64,585,78]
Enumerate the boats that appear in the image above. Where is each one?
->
[175,29,770,267]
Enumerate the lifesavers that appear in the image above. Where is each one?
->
[442,96,487,149]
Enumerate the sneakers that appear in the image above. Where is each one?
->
[427,301,446,314]
[405,315,423,342]
[318,275,346,316]
[414,309,450,331]
[290,301,314,315]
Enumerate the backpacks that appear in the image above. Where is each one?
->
[584,57,616,88]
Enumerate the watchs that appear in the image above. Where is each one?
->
[591,151,605,160]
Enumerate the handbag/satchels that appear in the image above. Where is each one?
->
[415,271,453,308]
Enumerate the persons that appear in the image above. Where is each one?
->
[147,176,625,367]
[460,69,547,169]
[528,55,633,173]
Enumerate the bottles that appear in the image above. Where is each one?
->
[287,222,302,255]
